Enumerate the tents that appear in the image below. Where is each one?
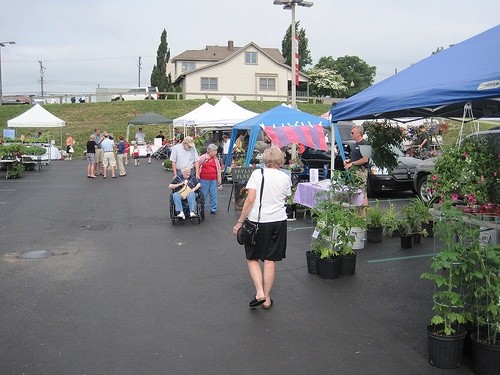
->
[173,96,260,138]
[126,112,172,143]
[331,25,500,185]
[221,105,350,179]
[7,103,66,158]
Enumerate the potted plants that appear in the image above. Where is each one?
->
[306,162,500,375]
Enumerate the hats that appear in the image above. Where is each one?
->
[185,135,196,147]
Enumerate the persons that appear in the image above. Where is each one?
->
[174,128,184,143]
[168,136,221,219]
[156,131,165,142]
[146,144,152,164]
[233,148,292,309]
[35,131,43,138]
[344,125,370,220]
[418,125,430,160]
[65,133,73,160]
[132,144,139,165]
[86,129,130,177]
[212,130,249,165]
[135,128,146,143]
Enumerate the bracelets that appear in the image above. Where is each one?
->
[238,219,244,224]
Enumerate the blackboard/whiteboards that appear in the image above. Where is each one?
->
[232,167,260,210]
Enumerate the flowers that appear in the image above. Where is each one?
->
[430,136,500,216]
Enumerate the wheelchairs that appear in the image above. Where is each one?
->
[169,188,205,225]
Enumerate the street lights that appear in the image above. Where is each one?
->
[0,41,16,105]
[273,0,315,107]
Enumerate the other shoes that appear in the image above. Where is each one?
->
[210,211,217,214]
[177,214,185,220]
[87,173,126,179]
[189,213,196,217]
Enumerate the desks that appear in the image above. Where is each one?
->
[22,155,42,172]
[295,180,368,220]
[0,159,16,179]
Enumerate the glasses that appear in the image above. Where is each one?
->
[350,132,357,135]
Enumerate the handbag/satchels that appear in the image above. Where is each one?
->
[242,218,259,246]
[178,184,192,199]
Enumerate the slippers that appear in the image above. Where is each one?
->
[249,295,266,307]
[262,299,273,309]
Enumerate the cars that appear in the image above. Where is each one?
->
[412,125,500,208]
[111,86,160,101]
[301,139,423,198]
